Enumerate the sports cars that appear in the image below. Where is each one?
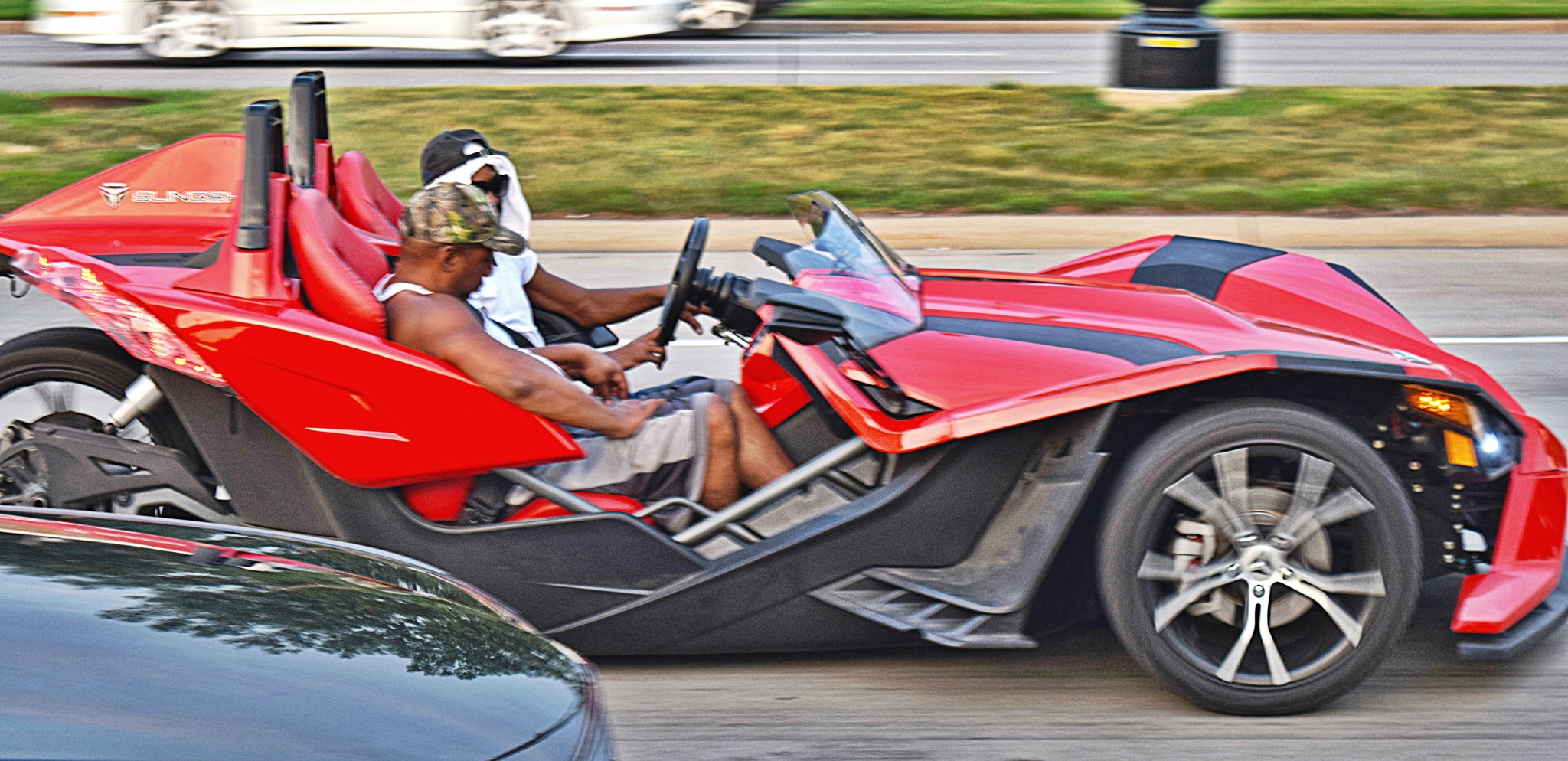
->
[0,70,1568,715]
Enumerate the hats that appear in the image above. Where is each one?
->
[396,181,526,256]
[419,129,511,186]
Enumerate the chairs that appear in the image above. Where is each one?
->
[335,151,619,353]
[286,188,654,528]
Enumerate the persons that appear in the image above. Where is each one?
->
[384,182,794,533]
[420,129,708,398]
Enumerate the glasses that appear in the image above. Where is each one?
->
[469,174,510,197]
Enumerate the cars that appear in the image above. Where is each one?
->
[0,496,617,761]
[26,0,759,62]
